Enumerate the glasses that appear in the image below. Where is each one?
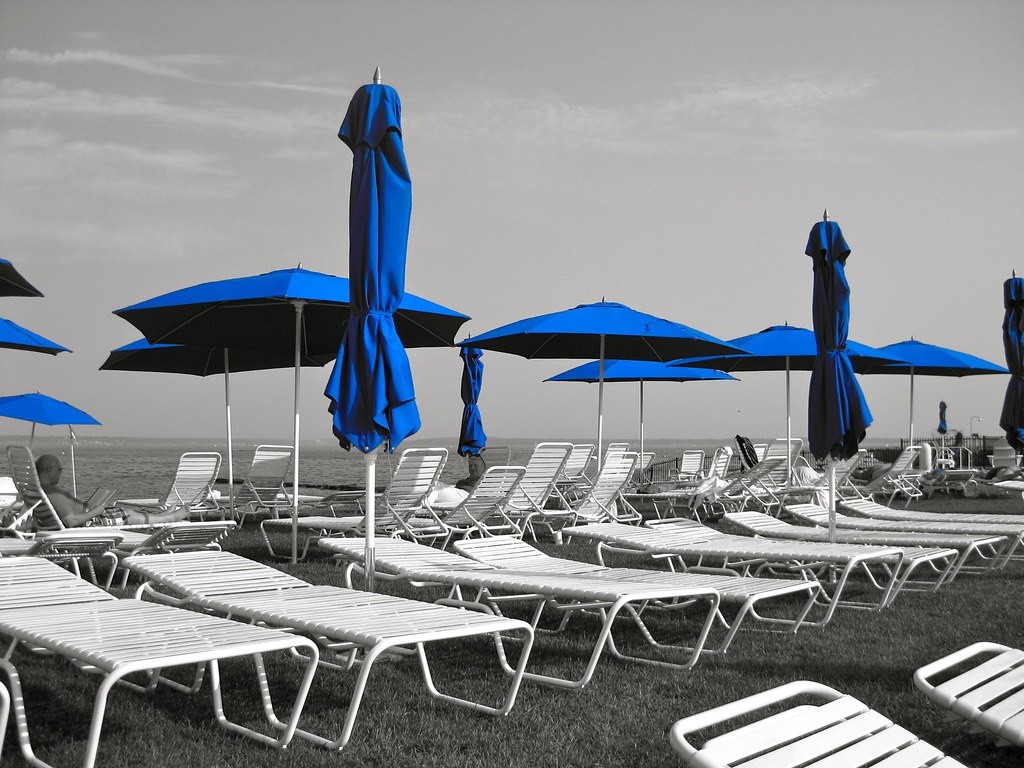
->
[51,466,62,472]
[469,454,486,468]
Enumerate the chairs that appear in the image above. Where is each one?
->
[0,430,1024,767]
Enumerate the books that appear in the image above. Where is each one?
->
[87,487,118,508]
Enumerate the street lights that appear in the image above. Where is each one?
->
[970,416,982,437]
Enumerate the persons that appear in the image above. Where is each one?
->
[424,454,487,505]
[975,464,1024,483]
[35,455,190,528]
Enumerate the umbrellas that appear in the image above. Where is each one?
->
[324,81,422,593]
[0,318,73,356]
[0,258,46,297]
[98,208,1024,582]
[0,390,102,452]
